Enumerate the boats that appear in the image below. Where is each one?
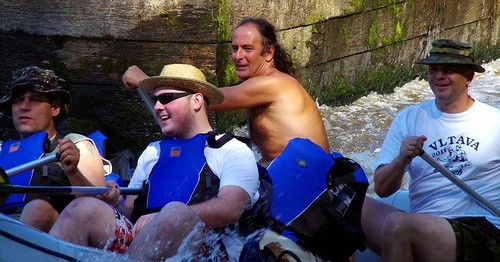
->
[0,192,497,261]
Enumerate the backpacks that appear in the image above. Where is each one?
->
[216,131,369,261]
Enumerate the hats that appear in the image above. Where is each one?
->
[414,38,486,73]
[0,66,73,113]
[139,64,225,106]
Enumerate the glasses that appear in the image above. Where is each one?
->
[151,92,191,104]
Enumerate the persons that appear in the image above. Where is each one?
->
[47,64,260,262]
[0,66,106,216]
[122,18,331,163]
[359,39,500,262]
